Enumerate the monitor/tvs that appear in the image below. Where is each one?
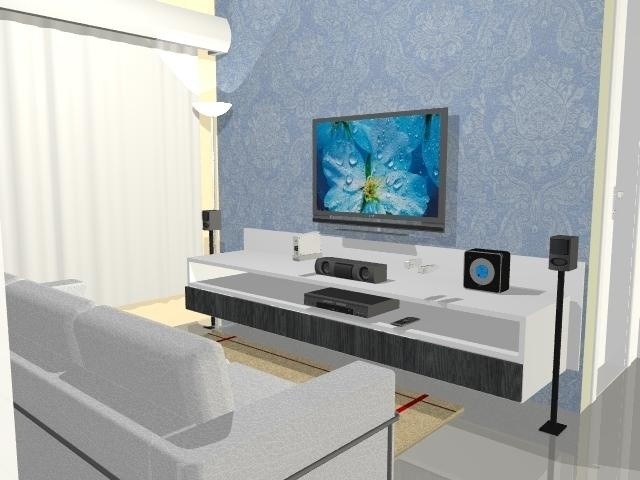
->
[311,106,448,234]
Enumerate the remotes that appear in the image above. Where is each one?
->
[392,316,420,326]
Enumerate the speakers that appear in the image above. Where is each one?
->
[463,247,510,293]
[548,235,579,270]
[313,256,388,285]
[202,210,223,230]
